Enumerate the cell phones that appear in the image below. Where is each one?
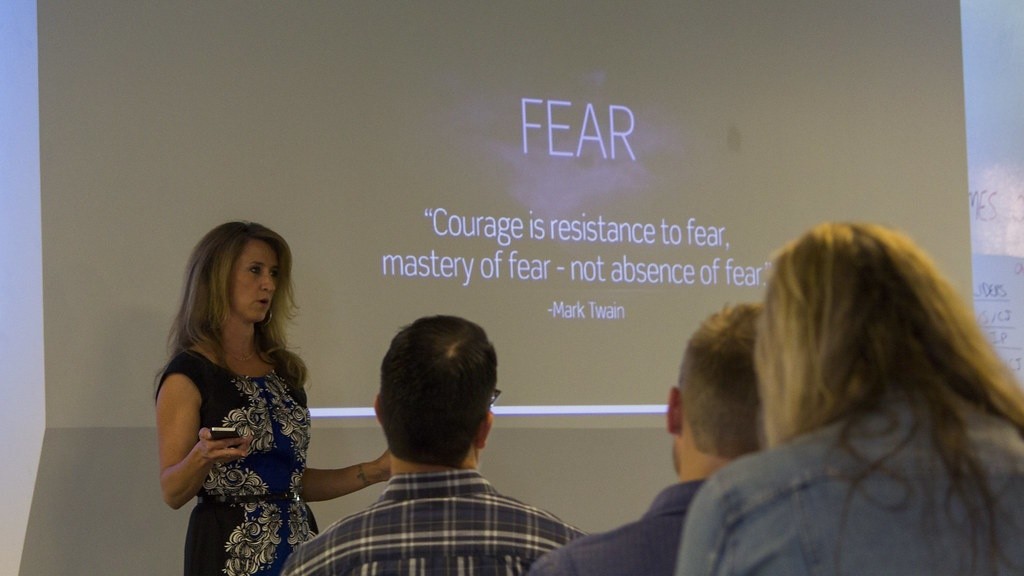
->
[211,427,241,449]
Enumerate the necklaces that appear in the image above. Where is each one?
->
[224,350,257,360]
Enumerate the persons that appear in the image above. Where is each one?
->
[522,304,761,576]
[669,220,1024,576]
[279,316,589,575]
[153,220,392,576]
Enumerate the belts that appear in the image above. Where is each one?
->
[197,491,305,507]
